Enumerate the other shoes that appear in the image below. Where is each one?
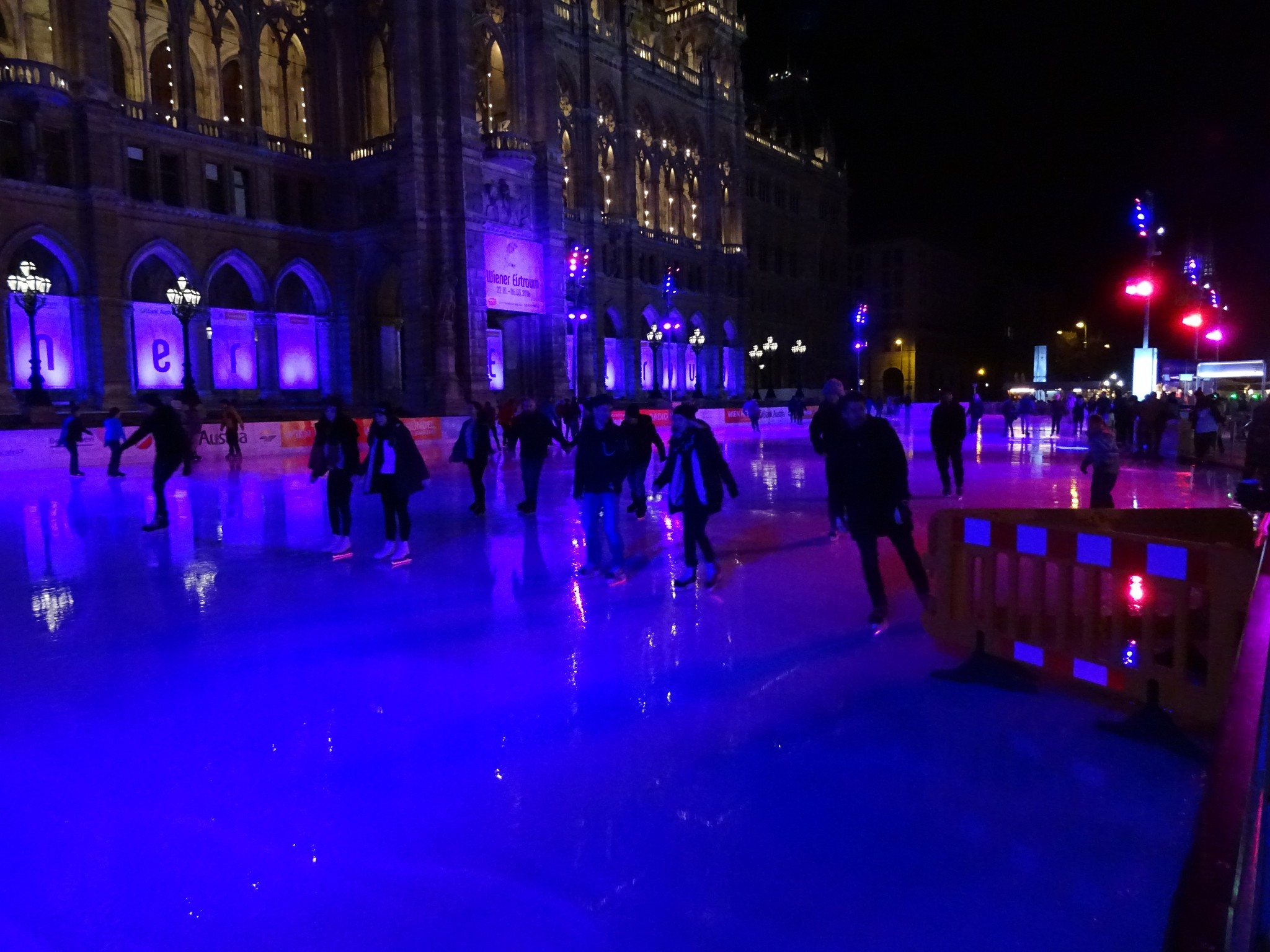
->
[196,455,201,459]
[69,470,84,477]
[142,518,169,533]
[320,538,351,553]
[627,504,647,518]
[943,484,951,496]
[955,485,964,495]
[470,503,484,514]
[225,452,242,458]
[828,529,839,540]
[517,499,535,512]
[109,471,125,477]
[868,606,888,624]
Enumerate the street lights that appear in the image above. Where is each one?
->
[895,338,904,371]
[567,310,587,401]
[853,344,866,392]
[688,328,707,399]
[6,260,53,407]
[1125,281,1152,348]
[791,339,807,388]
[1076,320,1088,349]
[1184,314,1202,362]
[646,322,663,397]
[166,274,202,406]
[748,344,763,399]
[1205,330,1222,361]
[761,335,778,399]
[661,322,680,402]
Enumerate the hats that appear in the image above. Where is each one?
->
[673,402,699,420]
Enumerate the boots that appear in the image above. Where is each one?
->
[389,541,409,559]
[371,542,396,558]
[674,567,698,587]
[703,560,718,588]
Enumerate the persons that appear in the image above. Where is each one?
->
[1234,395,1270,512]
[103,407,128,477]
[448,392,739,592]
[790,379,930,622]
[359,402,430,558]
[308,395,360,552]
[1081,414,1120,509]
[742,395,761,431]
[58,407,89,476]
[930,390,967,496]
[173,378,202,476]
[220,398,244,459]
[968,387,1261,466]
[113,391,186,532]
